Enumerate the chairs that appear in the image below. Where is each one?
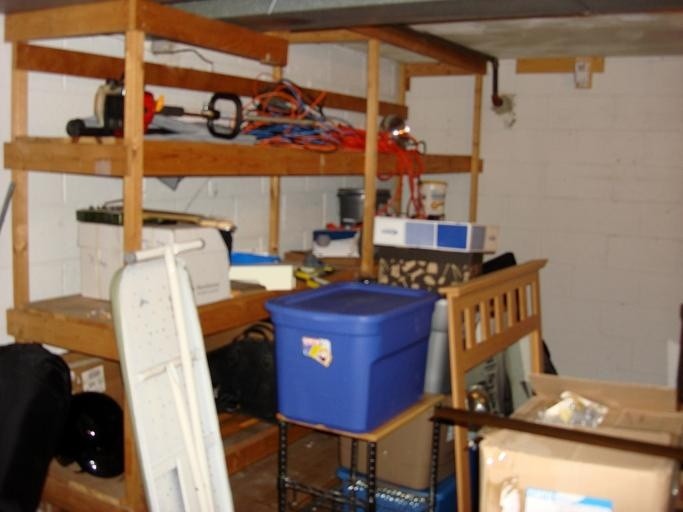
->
[421,296,470,399]
[437,259,683,507]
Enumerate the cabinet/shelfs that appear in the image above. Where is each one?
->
[268,400,447,512]
[8,2,491,512]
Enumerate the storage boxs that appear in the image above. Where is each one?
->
[481,367,680,511]
[273,277,431,423]
[372,217,486,252]
[329,464,460,509]
[338,391,458,489]
[362,291,479,398]
[376,255,486,299]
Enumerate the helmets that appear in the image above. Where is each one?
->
[49,392,124,478]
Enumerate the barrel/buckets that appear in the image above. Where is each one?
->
[336,187,389,224]
